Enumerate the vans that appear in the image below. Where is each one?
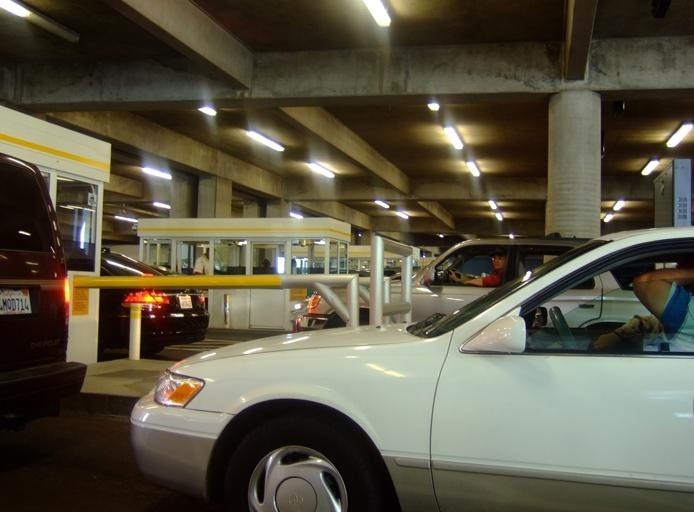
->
[0,149,88,433]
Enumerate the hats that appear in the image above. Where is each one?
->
[489,247,507,257]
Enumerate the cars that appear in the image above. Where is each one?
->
[119,219,694,512]
[99,248,211,360]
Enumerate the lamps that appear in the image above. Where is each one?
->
[246,129,285,152]
[198,106,217,116]
[289,211,303,218]
[640,156,660,176]
[488,199,498,210]
[396,211,408,220]
[444,124,464,149]
[427,101,440,112]
[466,159,481,177]
[665,122,693,148]
[613,199,625,212]
[307,161,335,178]
[0,1,80,45]
[603,212,613,222]
[375,199,390,208]
[495,211,503,221]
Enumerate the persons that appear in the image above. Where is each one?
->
[253,258,272,274]
[290,258,301,274]
[192,247,209,275]
[449,251,506,287]
[595,268,694,353]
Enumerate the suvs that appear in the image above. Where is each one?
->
[286,226,666,335]
